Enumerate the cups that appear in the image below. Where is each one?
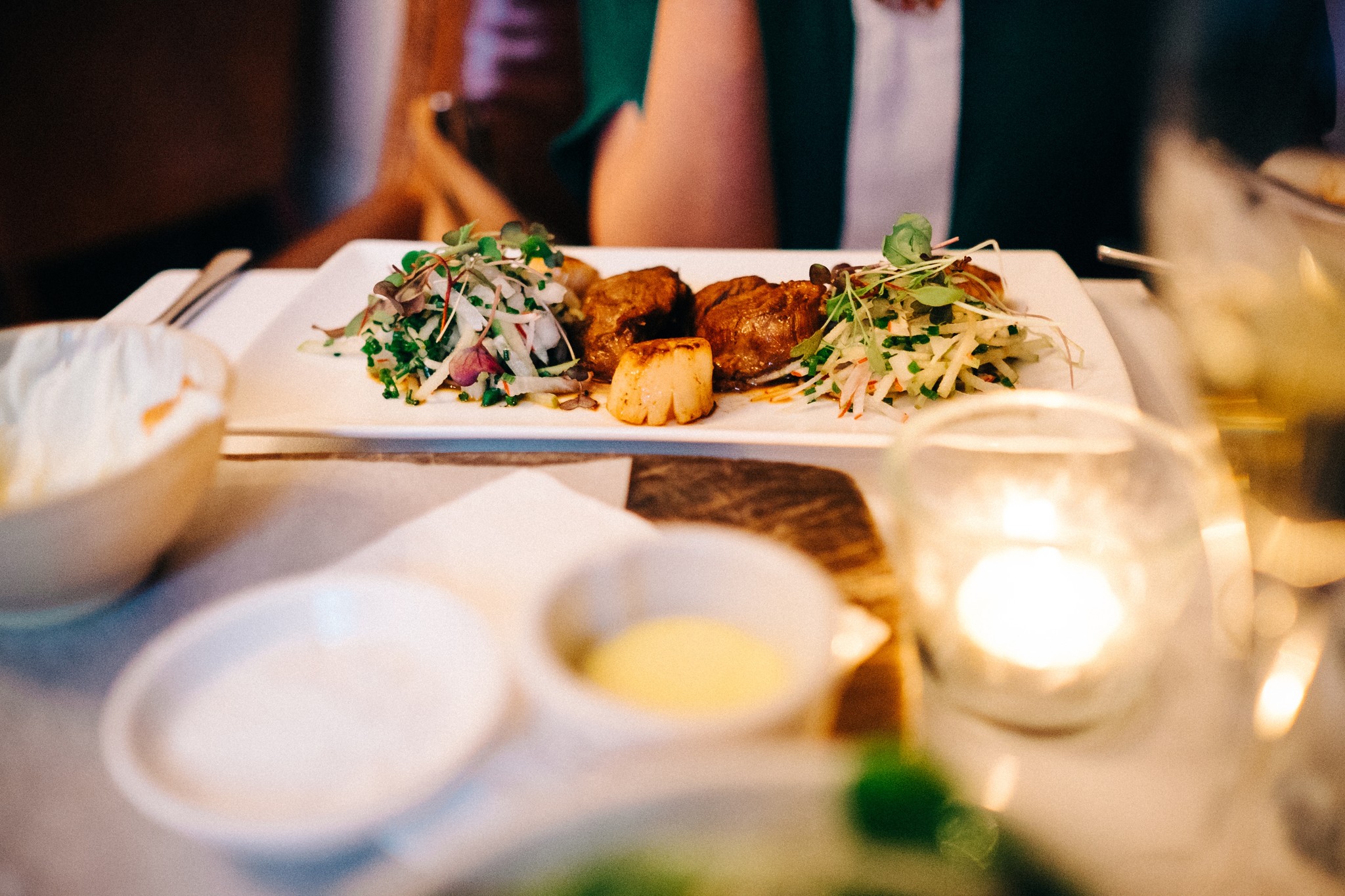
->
[1140,1,1343,581]
[346,750,1100,895]
[880,393,1210,738]
[514,514,840,759]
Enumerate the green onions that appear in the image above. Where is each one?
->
[802,299,1014,402]
[328,271,554,406]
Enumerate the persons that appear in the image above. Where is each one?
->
[461,0,1344,278]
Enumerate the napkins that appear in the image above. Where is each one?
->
[1,448,647,895]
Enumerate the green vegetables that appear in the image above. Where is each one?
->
[790,217,1002,366]
[343,219,564,337]
[515,759,1090,894]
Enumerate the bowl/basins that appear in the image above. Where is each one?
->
[99,569,510,880]
[1,315,234,631]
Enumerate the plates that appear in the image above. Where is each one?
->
[218,234,1145,471]
